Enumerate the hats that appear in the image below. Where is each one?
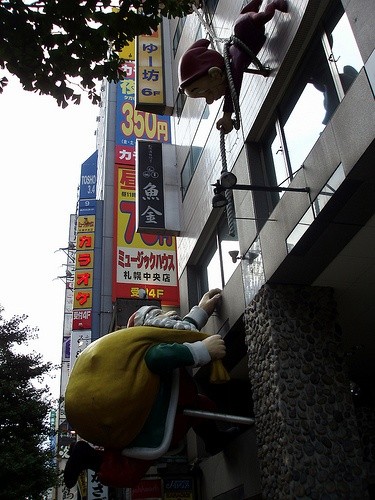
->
[177,39,225,93]
[125,306,160,328]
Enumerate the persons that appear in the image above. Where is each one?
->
[62,287,231,490]
[176,0,288,136]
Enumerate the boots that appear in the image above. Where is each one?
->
[64,441,104,489]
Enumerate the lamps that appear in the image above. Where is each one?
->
[209,171,309,211]
